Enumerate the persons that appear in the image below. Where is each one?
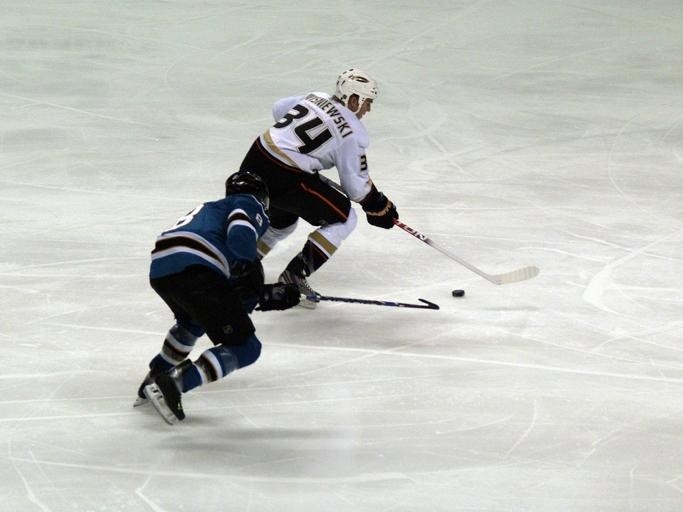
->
[239,66,401,309]
[135,172,303,424]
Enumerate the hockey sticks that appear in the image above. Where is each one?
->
[300,296,440,310]
[318,173,540,285]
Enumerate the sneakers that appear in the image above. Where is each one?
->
[154,371,185,421]
[278,268,321,303]
[137,382,148,400]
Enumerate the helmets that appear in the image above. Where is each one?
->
[335,67,379,108]
[225,171,269,212]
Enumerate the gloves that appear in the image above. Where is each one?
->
[228,257,264,301]
[361,191,397,229]
[258,282,300,311]
